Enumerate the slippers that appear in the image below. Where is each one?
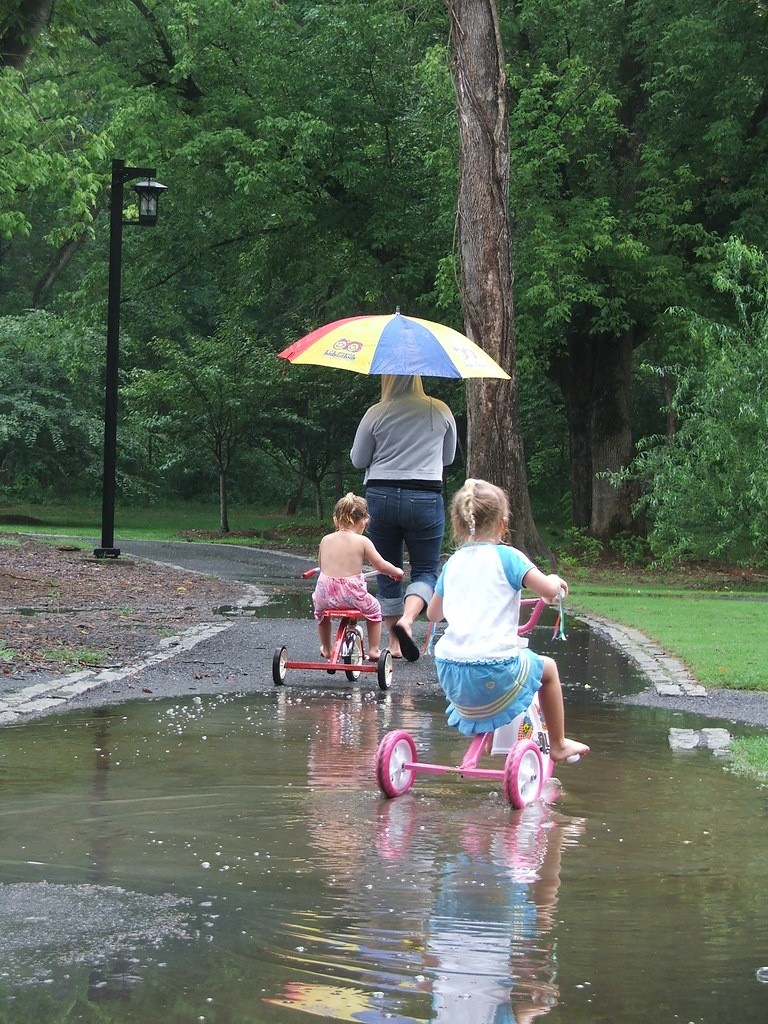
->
[391,624,420,661]
[386,647,401,659]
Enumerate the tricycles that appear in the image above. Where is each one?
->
[273,567,404,690]
[375,587,580,811]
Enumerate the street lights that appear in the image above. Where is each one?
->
[92,161,172,561]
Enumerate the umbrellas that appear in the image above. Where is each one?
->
[277,306,511,379]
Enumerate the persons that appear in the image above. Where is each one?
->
[312,493,404,658]
[350,374,457,661]
[428,479,589,761]
